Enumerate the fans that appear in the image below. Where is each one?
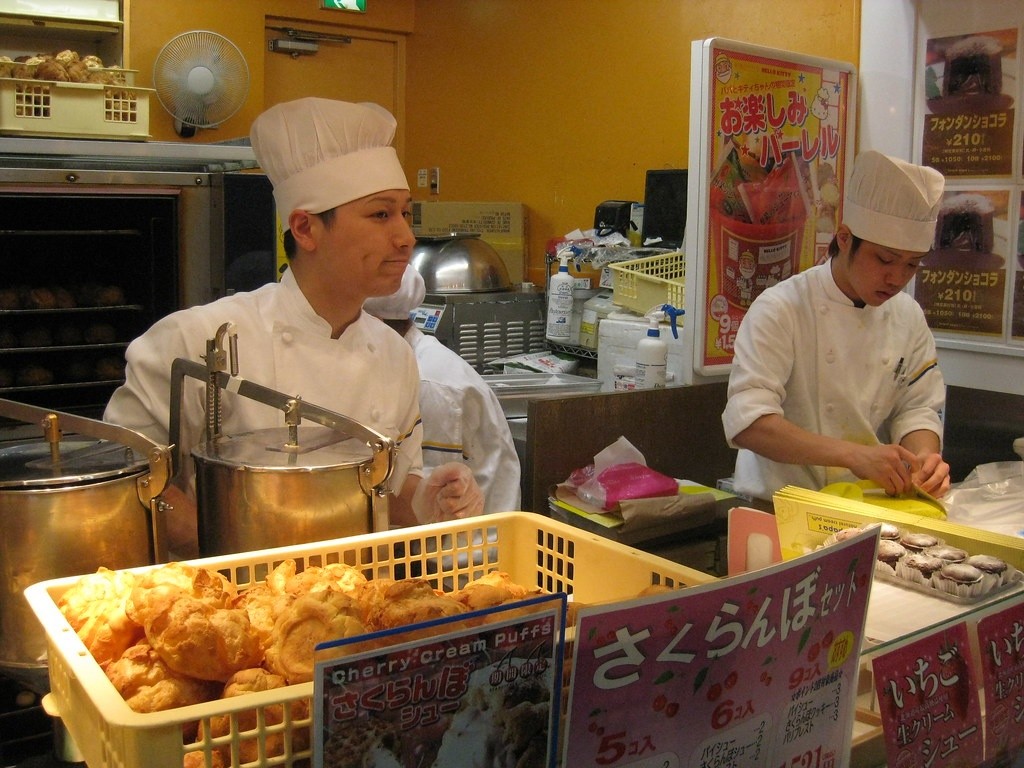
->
[153,29,249,138]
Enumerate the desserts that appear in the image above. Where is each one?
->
[825,521,1016,602]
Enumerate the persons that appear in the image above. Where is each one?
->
[102,97,486,584]
[362,263,521,594]
[721,151,951,503]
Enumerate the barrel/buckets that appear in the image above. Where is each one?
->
[709,187,809,315]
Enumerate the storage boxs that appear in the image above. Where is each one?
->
[413,200,530,281]
[607,253,690,326]
[24,511,731,767]
[596,310,685,383]
[0,62,157,142]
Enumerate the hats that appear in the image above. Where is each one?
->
[843,147,946,251]
[249,103,412,235]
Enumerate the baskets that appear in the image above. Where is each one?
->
[0,62,155,139]
[24,511,723,768]
[607,254,687,326]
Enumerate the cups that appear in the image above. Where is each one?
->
[934,208,995,253]
[943,53,1002,95]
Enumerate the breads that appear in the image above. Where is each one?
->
[0,49,137,121]
[66,560,673,768]
[0,279,127,388]
